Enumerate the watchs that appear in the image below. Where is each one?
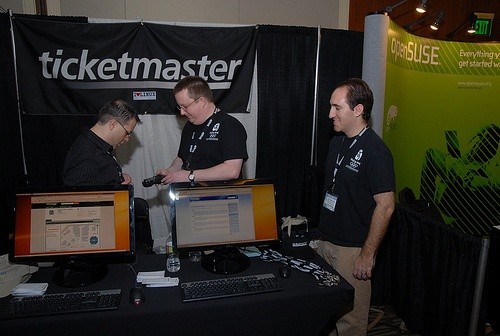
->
[188,169,195,183]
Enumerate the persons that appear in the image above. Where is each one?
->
[314,78,396,336]
[63,97,142,186]
[155,75,249,184]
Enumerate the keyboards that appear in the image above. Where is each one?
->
[180,273,284,304]
[2,289,120,318]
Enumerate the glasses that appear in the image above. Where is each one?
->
[177,96,204,112]
[113,117,133,138]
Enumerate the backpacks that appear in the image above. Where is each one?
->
[281,214,309,237]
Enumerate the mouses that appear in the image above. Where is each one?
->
[279,266,292,279]
[128,287,145,304]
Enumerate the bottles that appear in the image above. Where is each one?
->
[166,231,180,273]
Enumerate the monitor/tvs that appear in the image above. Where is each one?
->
[170,180,284,272]
[8,185,138,290]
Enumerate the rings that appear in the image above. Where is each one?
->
[361,271,367,275]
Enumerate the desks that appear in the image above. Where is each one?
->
[0,243,355,336]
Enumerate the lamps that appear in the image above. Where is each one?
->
[446,12,477,41]
[384,0,427,14]
[403,9,446,31]
